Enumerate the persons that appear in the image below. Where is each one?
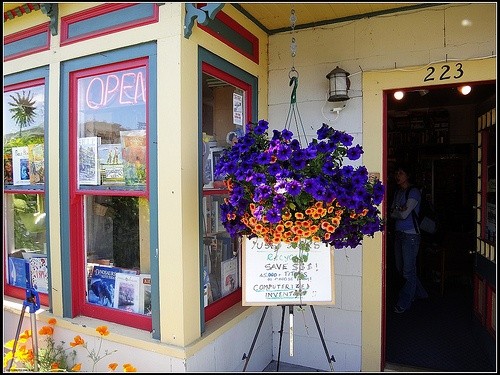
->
[388,160,423,314]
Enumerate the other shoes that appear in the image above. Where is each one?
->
[394,304,406,313]
[413,293,430,300]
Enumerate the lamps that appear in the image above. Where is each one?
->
[326,65,351,102]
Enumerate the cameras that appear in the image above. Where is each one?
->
[394,201,400,211]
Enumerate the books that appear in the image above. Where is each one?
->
[0,91,245,325]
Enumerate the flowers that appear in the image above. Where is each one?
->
[213,120,384,250]
